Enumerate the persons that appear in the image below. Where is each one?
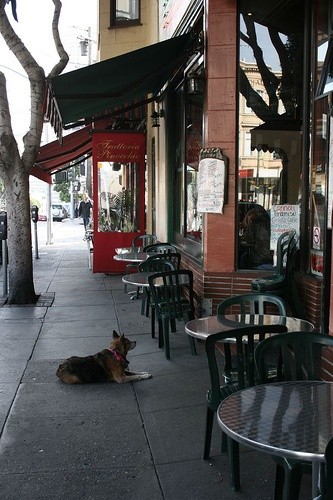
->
[239,208,275,273]
[79,193,93,231]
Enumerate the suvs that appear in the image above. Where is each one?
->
[51,203,68,221]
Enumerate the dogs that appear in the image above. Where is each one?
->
[55,329,152,386]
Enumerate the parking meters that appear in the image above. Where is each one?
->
[0,211,8,298]
[31,206,39,259]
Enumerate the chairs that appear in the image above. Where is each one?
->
[124,229,333,500]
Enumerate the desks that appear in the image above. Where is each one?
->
[217,380,333,500]
[115,246,176,255]
[122,271,196,288]
[113,253,169,264]
[185,313,314,344]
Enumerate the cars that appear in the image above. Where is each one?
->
[38,214,46,220]
